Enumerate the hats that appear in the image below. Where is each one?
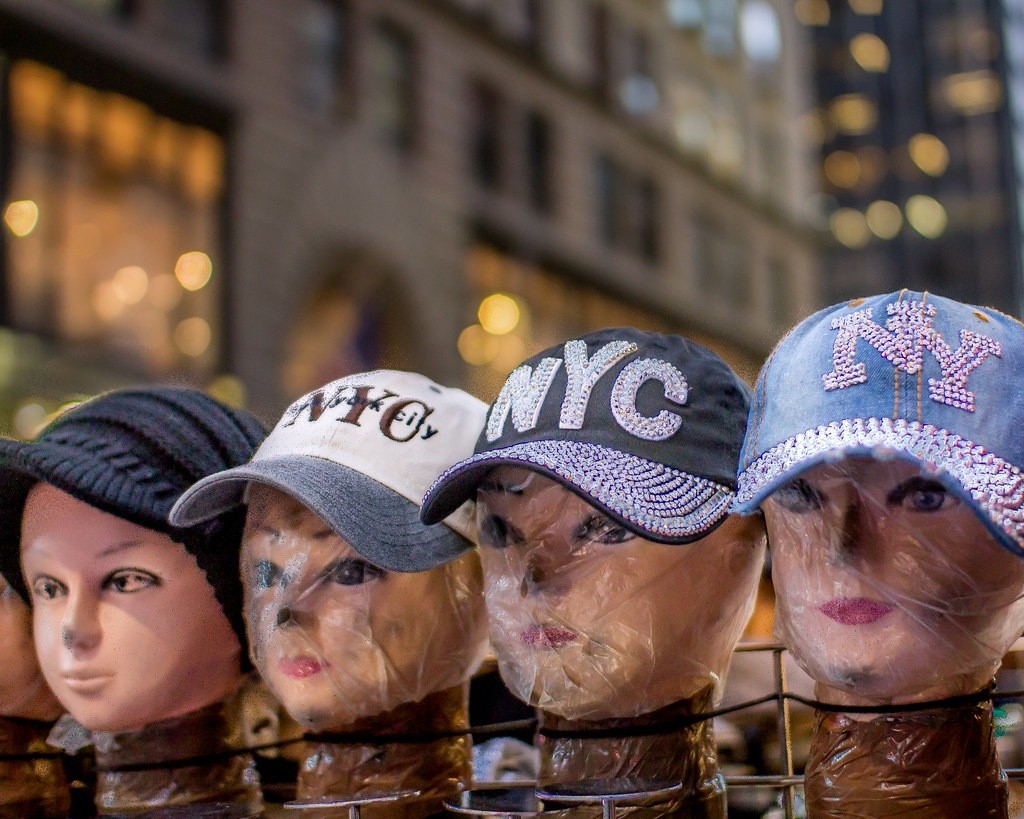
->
[727,288,1024,558]
[166,368,478,572]
[1,386,273,673]
[420,326,753,545]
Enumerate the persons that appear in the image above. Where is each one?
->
[169,369,490,806]
[735,288,1024,803]
[420,329,768,808]
[0,388,269,809]
